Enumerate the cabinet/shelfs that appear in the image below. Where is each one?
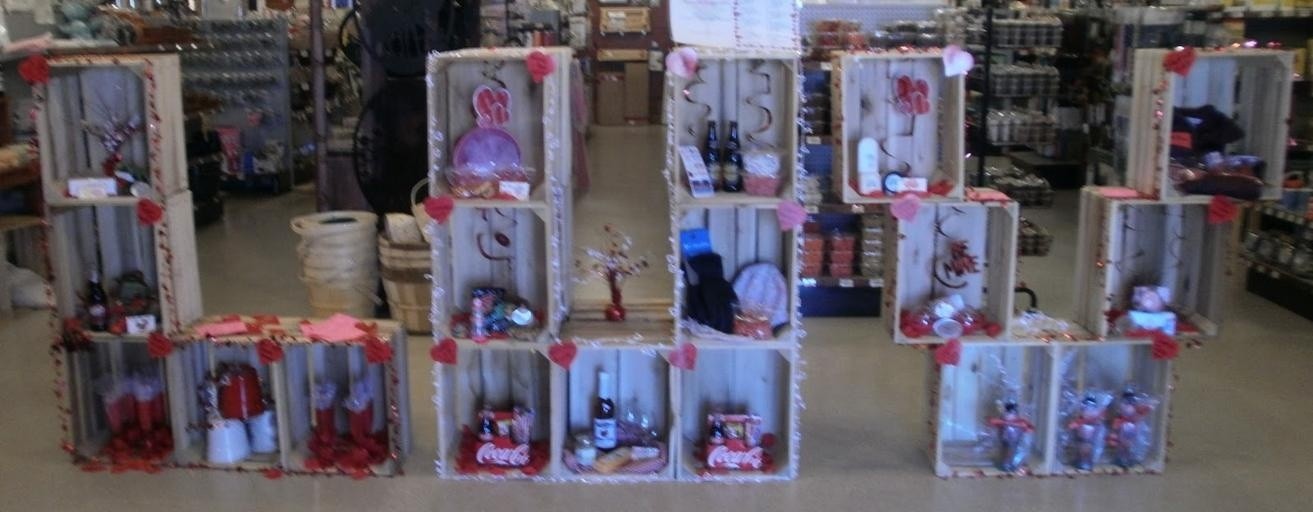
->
[0,1,1313,483]
